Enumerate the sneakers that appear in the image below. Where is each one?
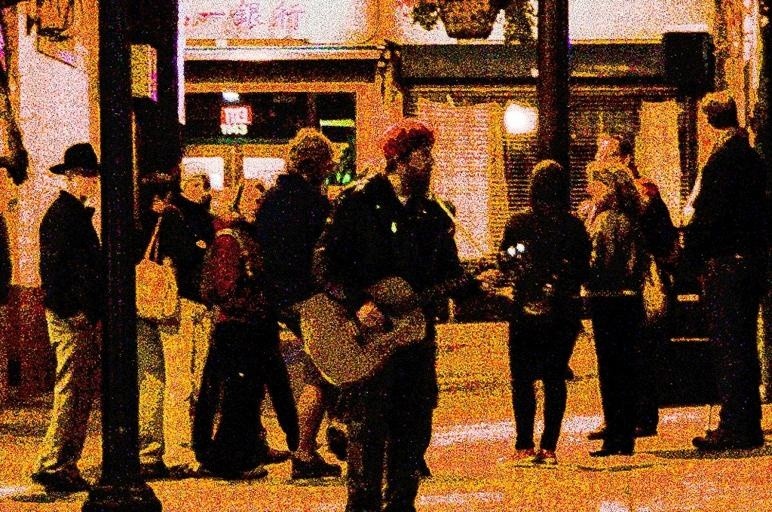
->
[232,446,341,479]
[497,449,559,466]
[692,425,764,449]
[587,423,656,456]
[30,468,92,490]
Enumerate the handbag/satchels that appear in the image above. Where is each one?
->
[641,255,669,327]
[134,255,178,322]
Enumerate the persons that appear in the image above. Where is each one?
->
[499,157,592,465]
[138,128,349,486]
[310,117,499,510]
[29,143,108,487]
[679,89,772,452]
[582,130,682,459]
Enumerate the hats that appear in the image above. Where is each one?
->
[375,118,435,157]
[50,143,102,174]
